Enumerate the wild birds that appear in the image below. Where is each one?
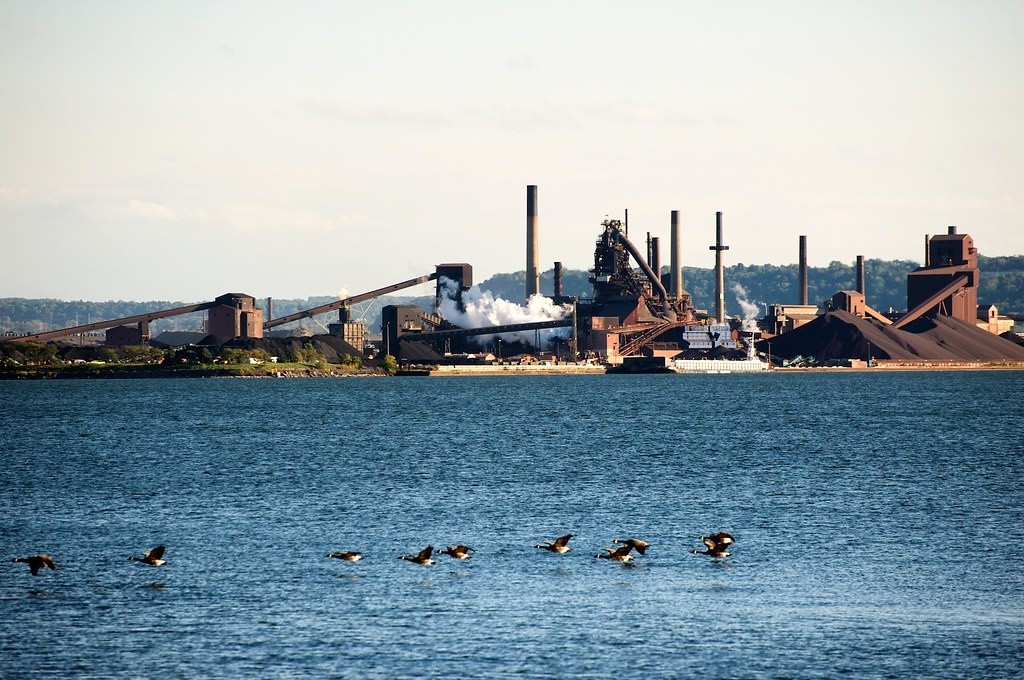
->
[396,544,437,567]
[594,538,652,564]
[690,532,735,563]
[325,550,364,563]
[534,534,573,554]
[435,543,475,560]
[127,545,171,567]
[9,554,56,575]
